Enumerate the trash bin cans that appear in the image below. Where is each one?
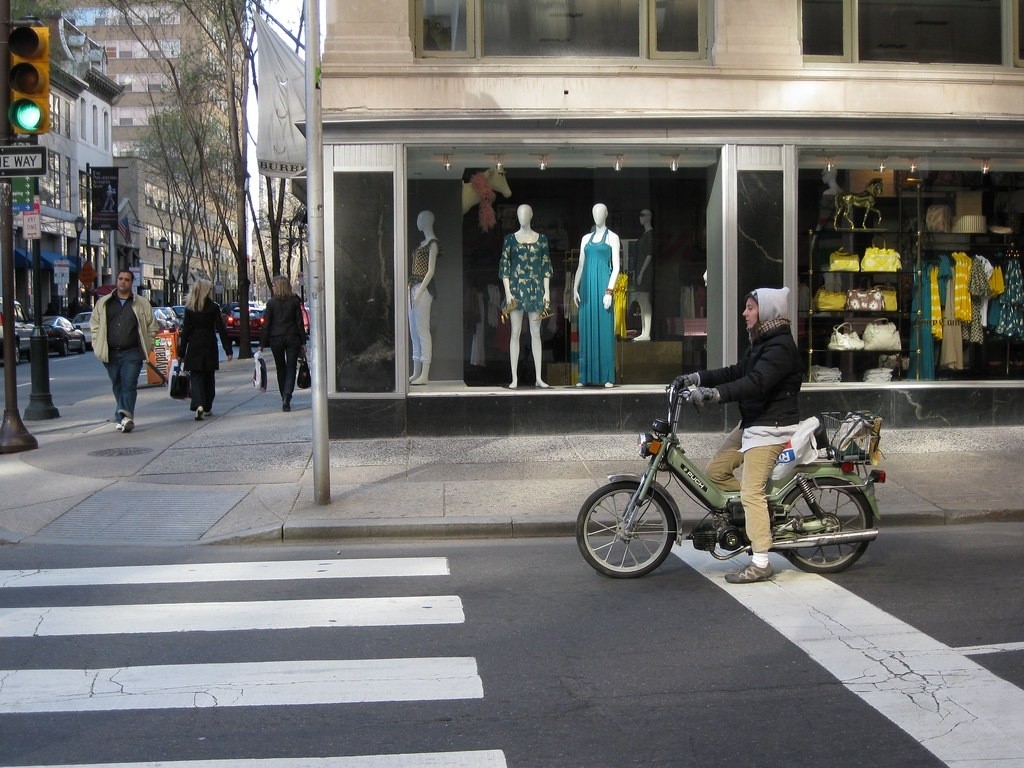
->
[147,326,180,387]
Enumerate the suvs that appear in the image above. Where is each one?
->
[0,297,35,366]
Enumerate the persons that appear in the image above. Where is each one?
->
[176,278,233,421]
[257,276,309,413]
[573,202,621,388]
[89,269,160,433]
[498,204,553,388]
[633,209,655,342]
[671,287,804,583]
[102,184,116,212]
[408,210,439,385]
[811,169,842,317]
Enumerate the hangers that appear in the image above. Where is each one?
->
[912,238,1020,263]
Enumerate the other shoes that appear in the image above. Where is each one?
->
[204,410,212,415]
[116,423,122,430]
[121,416,134,432]
[195,406,204,420]
[282,398,290,411]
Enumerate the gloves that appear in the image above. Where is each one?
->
[689,387,719,409]
[672,372,700,393]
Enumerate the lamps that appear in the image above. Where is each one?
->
[496,157,506,170]
[539,158,548,170]
[910,160,919,173]
[983,162,991,173]
[443,156,453,170]
[669,156,680,172]
[825,158,835,170]
[615,156,624,171]
[879,158,885,172]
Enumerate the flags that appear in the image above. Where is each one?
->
[252,8,308,178]
[118,215,132,244]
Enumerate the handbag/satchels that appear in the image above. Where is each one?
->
[297,349,311,388]
[815,229,903,351]
[831,413,875,461]
[252,350,267,391]
[171,358,189,399]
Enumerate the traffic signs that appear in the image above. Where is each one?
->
[0,145,47,176]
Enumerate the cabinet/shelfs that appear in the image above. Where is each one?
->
[808,172,920,379]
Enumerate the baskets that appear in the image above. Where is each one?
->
[823,411,882,461]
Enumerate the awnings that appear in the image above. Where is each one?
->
[171,266,211,284]
[38,250,76,272]
[67,255,86,273]
[15,247,44,270]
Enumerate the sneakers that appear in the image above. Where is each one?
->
[725,562,774,583]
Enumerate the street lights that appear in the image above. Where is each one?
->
[158,237,167,305]
[251,259,257,301]
[296,216,306,307]
[74,217,86,311]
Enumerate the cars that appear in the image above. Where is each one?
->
[221,303,266,347]
[31,316,85,356]
[72,312,93,351]
[152,306,186,336]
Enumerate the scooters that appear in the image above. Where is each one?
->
[575,383,887,578]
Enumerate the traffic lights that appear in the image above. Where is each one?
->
[7,26,51,135]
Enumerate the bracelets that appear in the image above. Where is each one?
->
[606,289,613,295]
